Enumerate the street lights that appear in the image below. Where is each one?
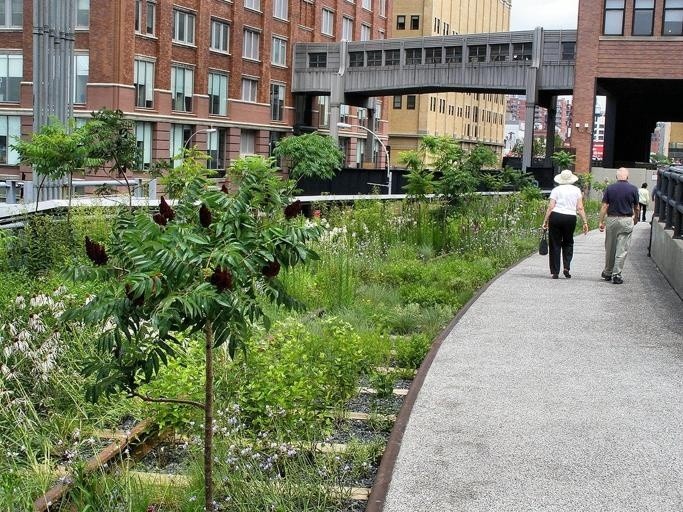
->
[180,127,216,178]
[334,122,392,195]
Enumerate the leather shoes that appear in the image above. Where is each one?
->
[613,276,624,284]
[552,273,558,279]
[601,271,611,281]
[563,268,571,278]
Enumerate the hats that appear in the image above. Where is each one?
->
[554,169,579,185]
[616,167,628,181]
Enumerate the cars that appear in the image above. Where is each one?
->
[591,156,597,162]
[646,152,682,168]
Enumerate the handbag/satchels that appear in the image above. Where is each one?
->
[539,228,548,255]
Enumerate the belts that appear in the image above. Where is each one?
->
[608,214,631,217]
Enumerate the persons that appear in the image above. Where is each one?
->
[649,185,657,225]
[638,183,649,222]
[542,169,589,279]
[599,167,640,284]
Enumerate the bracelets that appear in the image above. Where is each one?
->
[583,221,588,224]
